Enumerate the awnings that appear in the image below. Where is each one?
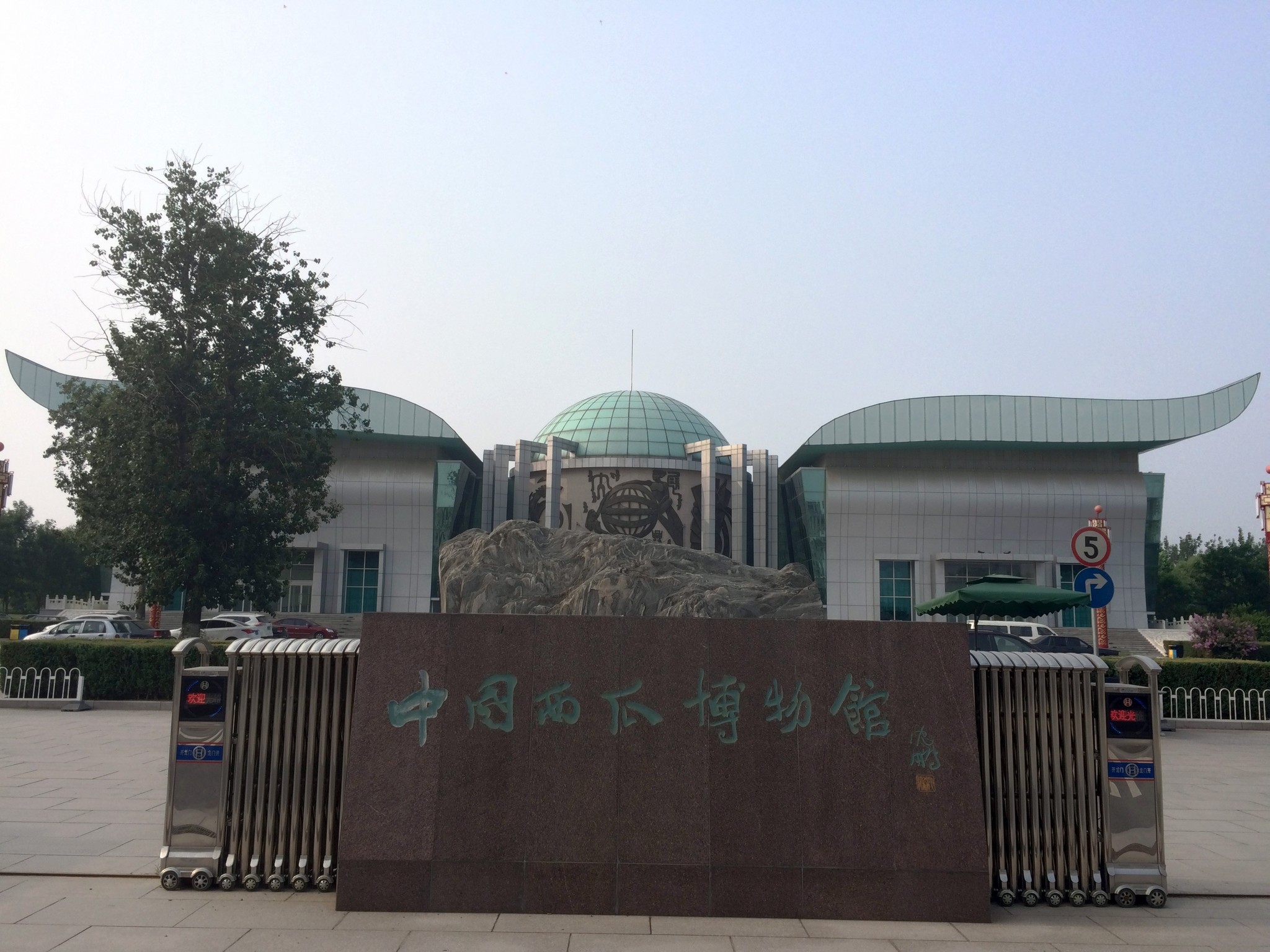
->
[914,572,1091,652]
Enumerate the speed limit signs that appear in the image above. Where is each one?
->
[1069,525,1113,568]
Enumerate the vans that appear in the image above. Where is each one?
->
[966,619,1059,643]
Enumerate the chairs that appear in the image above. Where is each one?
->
[82,623,97,633]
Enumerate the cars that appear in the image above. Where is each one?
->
[22,618,131,639]
[272,617,339,639]
[169,618,261,642]
[1028,635,1121,656]
[119,619,172,638]
[969,629,1051,652]
[0,613,131,633]
[273,623,288,639]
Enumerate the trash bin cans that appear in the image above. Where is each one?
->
[1168,645,1177,658]
[10,624,29,640]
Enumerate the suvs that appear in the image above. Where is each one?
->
[211,613,274,638]
